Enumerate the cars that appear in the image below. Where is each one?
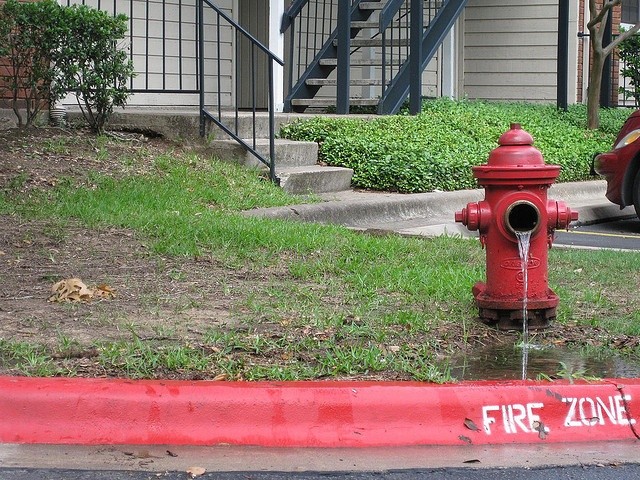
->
[594,107,639,218]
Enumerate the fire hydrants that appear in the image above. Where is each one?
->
[455,124,578,331]
